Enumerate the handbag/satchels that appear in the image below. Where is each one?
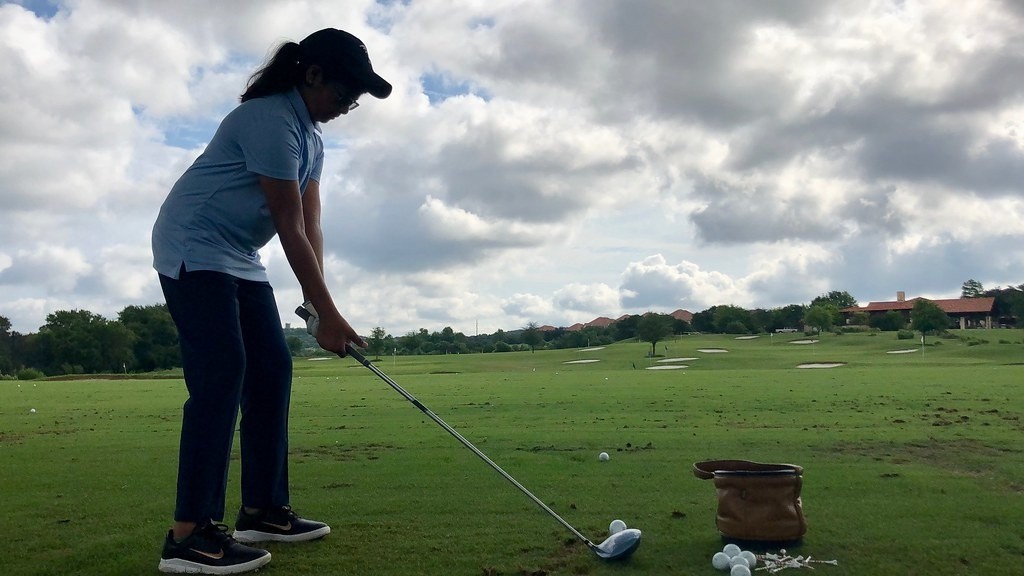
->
[692,459,806,541]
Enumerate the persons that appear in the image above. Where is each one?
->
[153,27,392,576]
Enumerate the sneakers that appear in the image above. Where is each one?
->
[232,503,330,542]
[158,520,272,575]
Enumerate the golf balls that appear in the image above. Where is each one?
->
[609,519,627,534]
[711,543,757,576]
[31,408,36,413]
[598,452,610,461]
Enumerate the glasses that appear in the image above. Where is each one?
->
[323,76,359,112]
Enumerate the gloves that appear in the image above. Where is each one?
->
[302,299,321,336]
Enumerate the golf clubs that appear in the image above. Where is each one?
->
[293,305,643,559]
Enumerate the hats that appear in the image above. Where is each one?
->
[300,28,392,98]
